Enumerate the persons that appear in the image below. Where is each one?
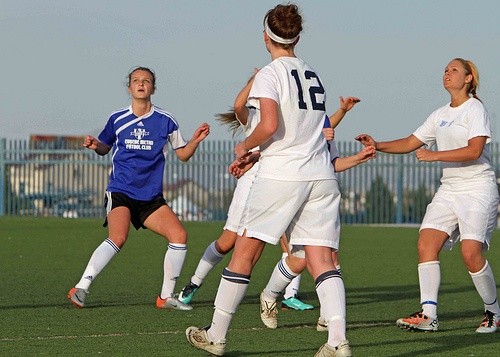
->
[186,2,355,357]
[66,64,210,311]
[355,57,500,335]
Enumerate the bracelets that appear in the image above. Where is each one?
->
[341,108,348,113]
[244,136,248,147]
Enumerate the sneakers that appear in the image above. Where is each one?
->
[178,276,202,304]
[185,326,226,357]
[67,288,90,308]
[316,317,328,331]
[314,340,352,357]
[156,294,193,311]
[260,292,278,329]
[475,310,500,333]
[396,309,439,332]
[281,297,314,311]
